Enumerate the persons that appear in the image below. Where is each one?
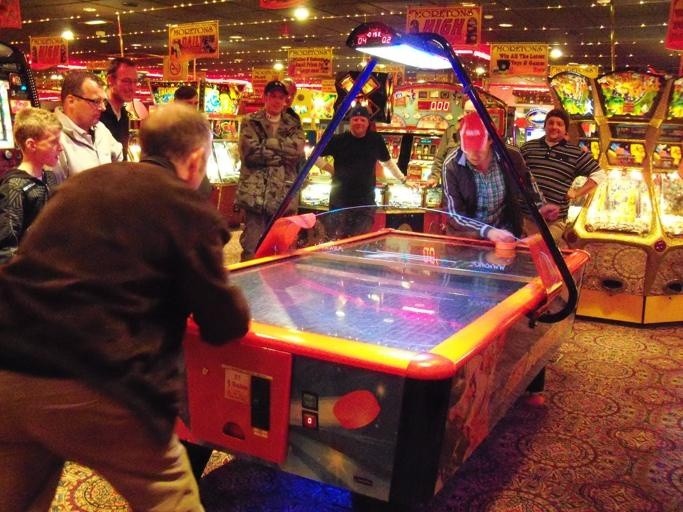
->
[422,101,477,191]
[516,106,605,250]
[436,235,526,330]
[234,78,310,263]
[0,99,249,512]
[441,111,561,248]
[1,57,138,250]
[314,106,420,242]
[174,86,199,110]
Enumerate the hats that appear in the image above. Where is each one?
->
[265,78,297,95]
[460,112,488,153]
[544,109,569,131]
[351,107,369,118]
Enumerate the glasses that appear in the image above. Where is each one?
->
[73,94,108,105]
[112,75,139,83]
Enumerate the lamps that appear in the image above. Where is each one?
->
[345,19,454,71]
